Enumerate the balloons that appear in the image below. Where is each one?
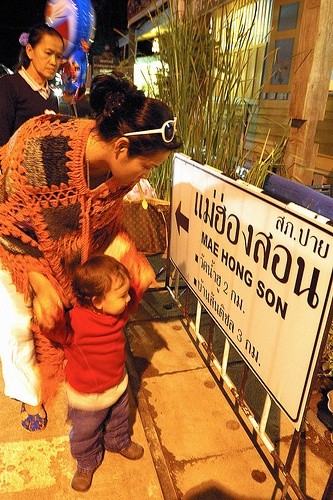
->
[45,0,96,103]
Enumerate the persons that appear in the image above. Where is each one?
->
[0,75,178,433]
[0,25,64,148]
[48,75,92,119]
[41,257,144,493]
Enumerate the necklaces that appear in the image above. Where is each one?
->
[85,134,111,190]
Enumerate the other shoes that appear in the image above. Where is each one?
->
[116,439,145,460]
[71,464,101,492]
[21,402,48,433]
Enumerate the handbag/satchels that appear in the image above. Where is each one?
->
[119,182,170,256]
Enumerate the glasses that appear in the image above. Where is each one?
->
[124,116,178,143]
